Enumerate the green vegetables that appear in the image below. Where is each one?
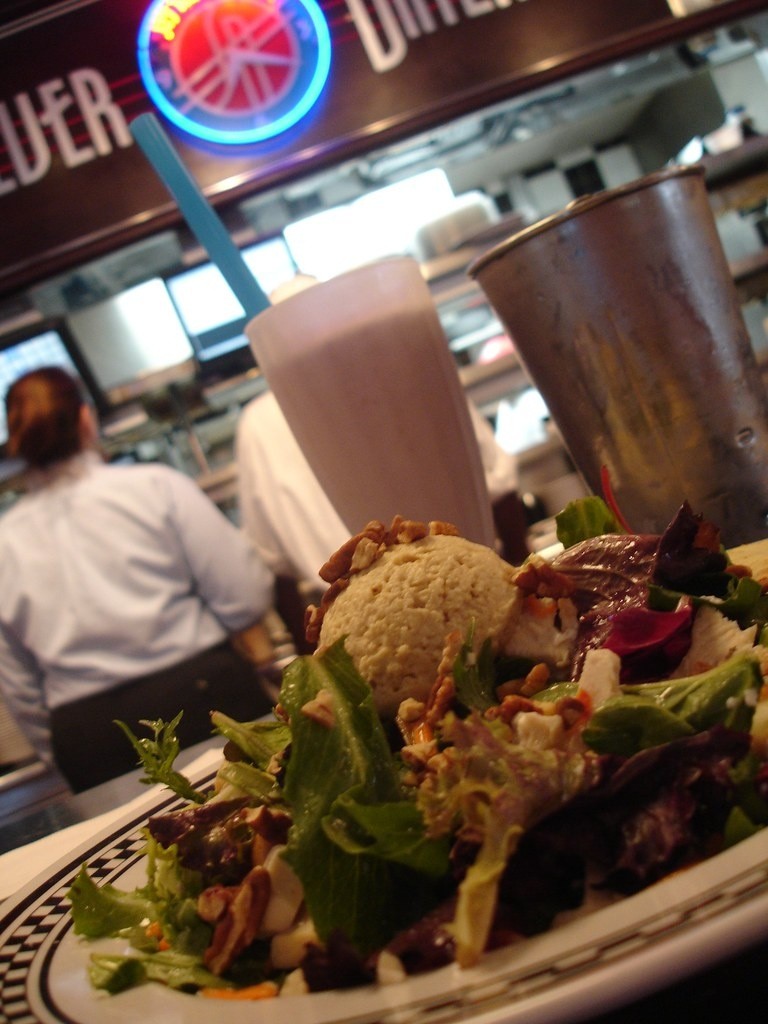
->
[555,496,626,549]
[70,631,758,993]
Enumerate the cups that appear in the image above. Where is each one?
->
[466,158,768,539]
[240,248,497,553]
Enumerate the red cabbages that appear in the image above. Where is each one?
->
[513,496,703,687]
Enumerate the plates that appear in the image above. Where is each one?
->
[0,739,768,1024]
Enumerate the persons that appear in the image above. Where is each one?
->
[235,280,529,610]
[1,366,268,792]
[476,333,560,456]
